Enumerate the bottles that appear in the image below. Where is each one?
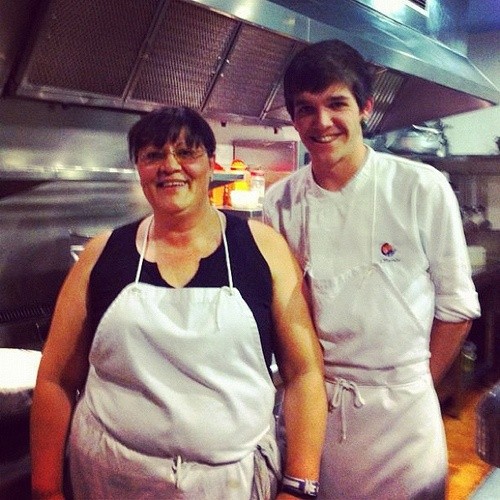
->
[251,169,267,211]
[230,158,249,210]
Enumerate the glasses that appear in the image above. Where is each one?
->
[136,145,211,164]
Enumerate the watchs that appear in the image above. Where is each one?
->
[281,476,319,499]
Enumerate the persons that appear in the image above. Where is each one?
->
[263,39,481,500]
[30,106,328,500]
